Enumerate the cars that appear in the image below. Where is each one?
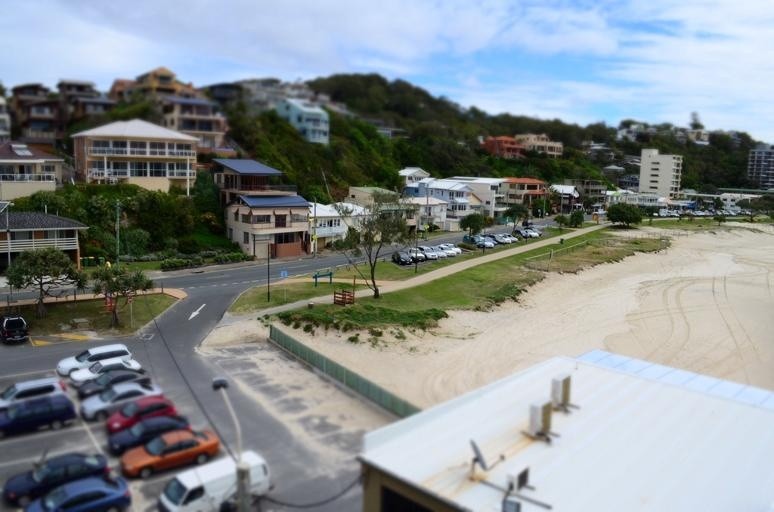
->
[5,453,132,512]
[57,342,220,476]
[463,233,518,248]
[512,227,542,238]
[666,208,752,216]
[392,242,462,264]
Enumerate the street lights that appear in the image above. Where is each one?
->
[212,375,253,512]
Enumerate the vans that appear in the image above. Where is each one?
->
[158,450,270,512]
[2,317,29,344]
[0,377,75,436]
